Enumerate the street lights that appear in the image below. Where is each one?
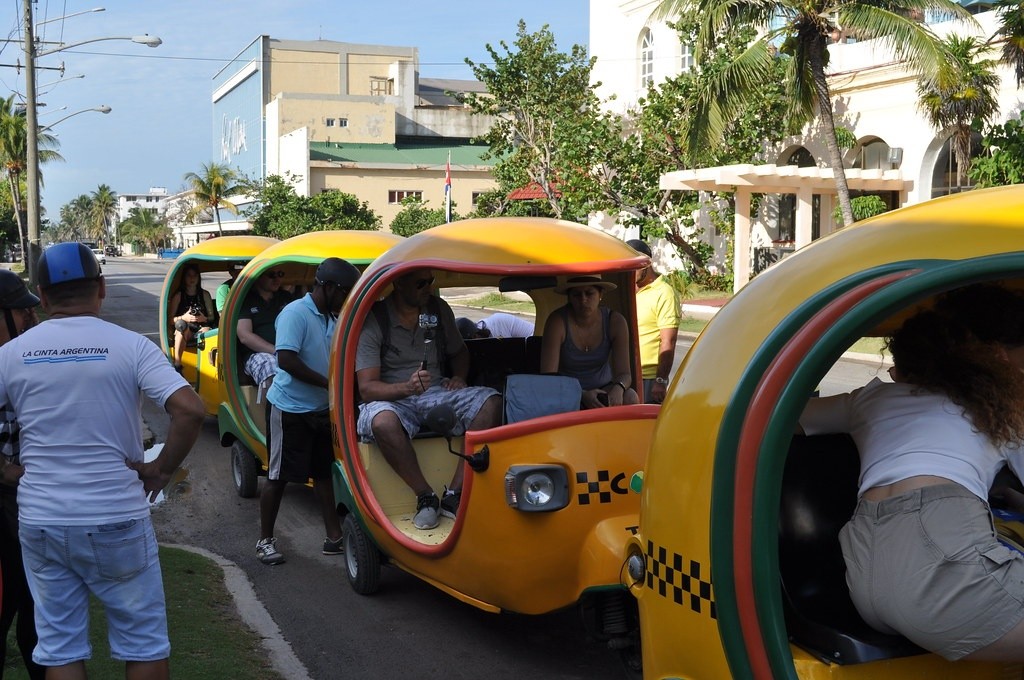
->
[25,6,161,295]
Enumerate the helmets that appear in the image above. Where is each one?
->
[314,258,361,287]
[626,240,652,259]
[1,270,41,310]
[36,241,102,286]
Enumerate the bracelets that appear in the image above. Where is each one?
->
[614,381,627,392]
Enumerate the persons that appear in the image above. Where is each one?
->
[157,246,164,260]
[236,265,296,406]
[793,310,1024,665]
[354,267,504,531]
[455,312,535,339]
[255,257,362,565]
[0,240,206,680]
[0,268,47,680]
[929,279,1024,373]
[625,239,682,405]
[167,265,215,374]
[6,246,16,263]
[215,261,248,319]
[534,273,640,410]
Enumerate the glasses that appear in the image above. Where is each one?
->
[402,277,436,289]
[263,271,285,279]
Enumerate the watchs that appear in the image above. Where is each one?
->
[655,377,668,384]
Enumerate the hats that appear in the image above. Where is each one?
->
[554,274,617,293]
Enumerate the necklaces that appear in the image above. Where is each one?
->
[573,310,601,352]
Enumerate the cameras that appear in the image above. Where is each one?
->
[418,312,440,329]
[185,300,202,317]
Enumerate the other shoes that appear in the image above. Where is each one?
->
[174,365,184,377]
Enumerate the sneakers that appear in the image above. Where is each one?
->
[256,539,284,565]
[323,537,346,555]
[412,493,441,529]
[440,484,461,522]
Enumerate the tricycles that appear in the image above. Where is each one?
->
[159,181,1024,679]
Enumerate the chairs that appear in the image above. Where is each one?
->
[529,336,547,377]
[445,338,527,393]
[777,434,939,666]
[236,336,260,388]
[169,299,221,347]
